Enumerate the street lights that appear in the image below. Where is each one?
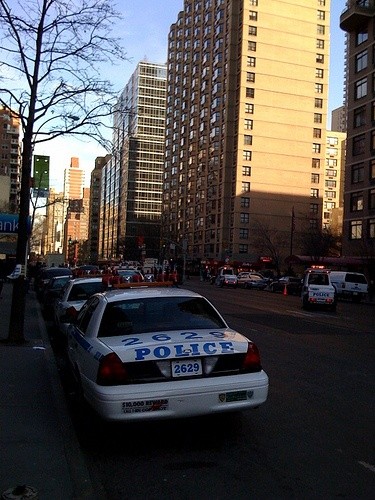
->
[32,115,80,153]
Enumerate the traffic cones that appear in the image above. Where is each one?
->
[282,284,287,296]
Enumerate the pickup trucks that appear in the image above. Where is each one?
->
[301,265,336,313]
[216,267,237,288]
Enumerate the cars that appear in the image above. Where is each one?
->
[32,267,76,302]
[270,276,302,292]
[112,268,144,284]
[54,277,111,323]
[65,271,270,422]
[237,271,269,290]
[72,265,100,277]
[43,275,78,313]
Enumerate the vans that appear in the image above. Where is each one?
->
[328,270,368,300]
